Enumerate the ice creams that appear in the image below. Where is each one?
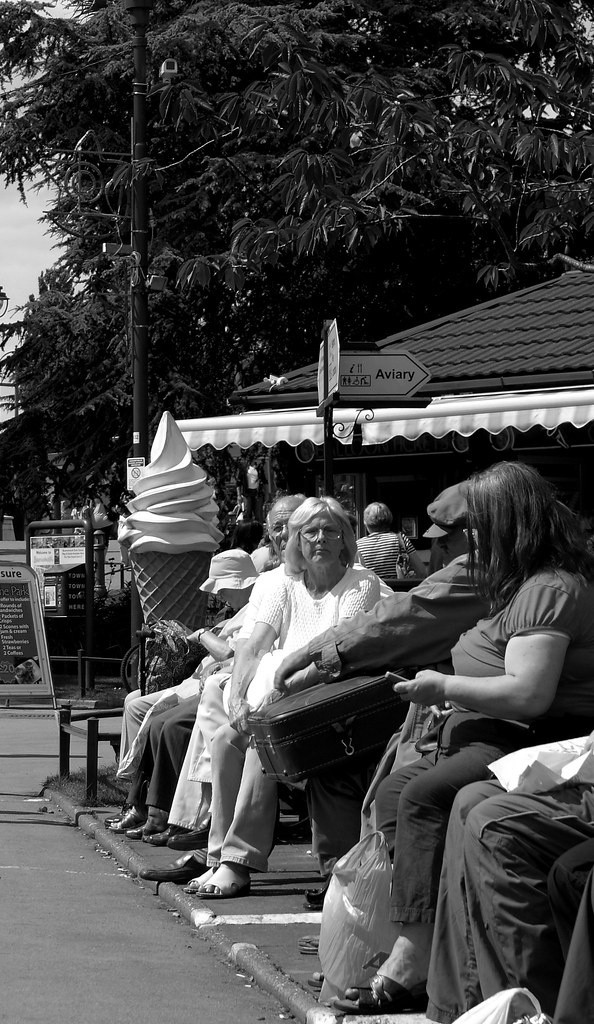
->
[117,410,226,633]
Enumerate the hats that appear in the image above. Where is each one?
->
[423,480,470,536]
[200,549,260,594]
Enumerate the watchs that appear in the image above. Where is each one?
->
[198,629,209,641]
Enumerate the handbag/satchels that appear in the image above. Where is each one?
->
[317,831,403,1003]
[395,553,415,579]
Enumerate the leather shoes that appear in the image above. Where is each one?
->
[302,874,332,910]
[139,852,209,882]
[143,824,192,846]
[105,812,125,828]
[109,808,147,832]
[125,825,149,840]
[167,818,210,851]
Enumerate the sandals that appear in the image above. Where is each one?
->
[333,971,428,1014]
[307,972,324,987]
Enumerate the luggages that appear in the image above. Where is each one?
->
[247,667,411,782]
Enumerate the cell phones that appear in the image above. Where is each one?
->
[385,671,410,684]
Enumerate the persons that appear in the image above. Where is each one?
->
[355,501,428,578]
[308,461,594,1024]
[104,483,503,957]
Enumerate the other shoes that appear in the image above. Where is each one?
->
[298,935,319,954]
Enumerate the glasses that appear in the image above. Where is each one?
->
[300,524,343,540]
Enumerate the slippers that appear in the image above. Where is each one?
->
[196,864,252,897]
[183,866,214,893]
